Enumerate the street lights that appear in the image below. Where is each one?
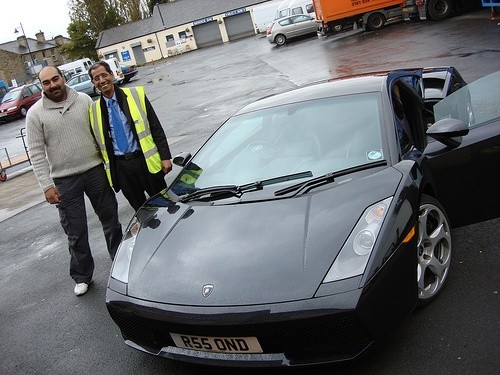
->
[13,22,35,66]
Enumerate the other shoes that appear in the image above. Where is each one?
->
[74,282,89,295]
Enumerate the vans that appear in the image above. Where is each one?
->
[274,0,316,20]
[0,83,44,124]
[102,57,126,86]
[54,57,93,82]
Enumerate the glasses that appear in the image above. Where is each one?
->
[92,71,110,81]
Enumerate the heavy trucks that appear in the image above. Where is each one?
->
[312,0,453,35]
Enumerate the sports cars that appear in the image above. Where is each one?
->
[105,65,500,375]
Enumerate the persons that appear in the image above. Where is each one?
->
[25,66,124,295]
[87,61,173,212]
[135,208,161,229]
[143,194,180,214]
[170,180,200,196]
[180,161,203,184]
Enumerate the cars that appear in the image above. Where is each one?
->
[265,14,318,46]
[119,64,138,83]
[65,72,119,98]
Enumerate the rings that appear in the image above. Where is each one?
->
[51,202,52,203]
